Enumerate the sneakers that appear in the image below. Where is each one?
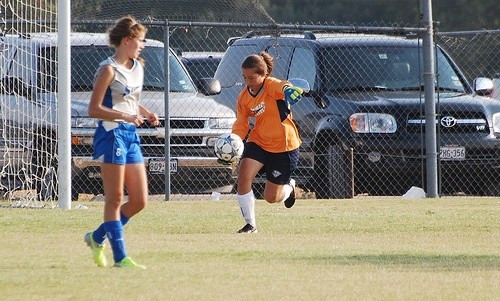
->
[84,232,105,267]
[112,256,145,271]
[237,224,257,233]
[284,178,295,208]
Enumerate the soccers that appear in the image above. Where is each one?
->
[214,133,245,163]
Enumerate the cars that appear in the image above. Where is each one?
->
[483,76,500,102]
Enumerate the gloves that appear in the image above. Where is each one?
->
[285,86,303,107]
[217,158,232,165]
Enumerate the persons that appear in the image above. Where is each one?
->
[216,50,306,234]
[85,16,161,270]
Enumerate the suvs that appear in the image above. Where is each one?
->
[0,31,237,202]
[211,28,500,198]
